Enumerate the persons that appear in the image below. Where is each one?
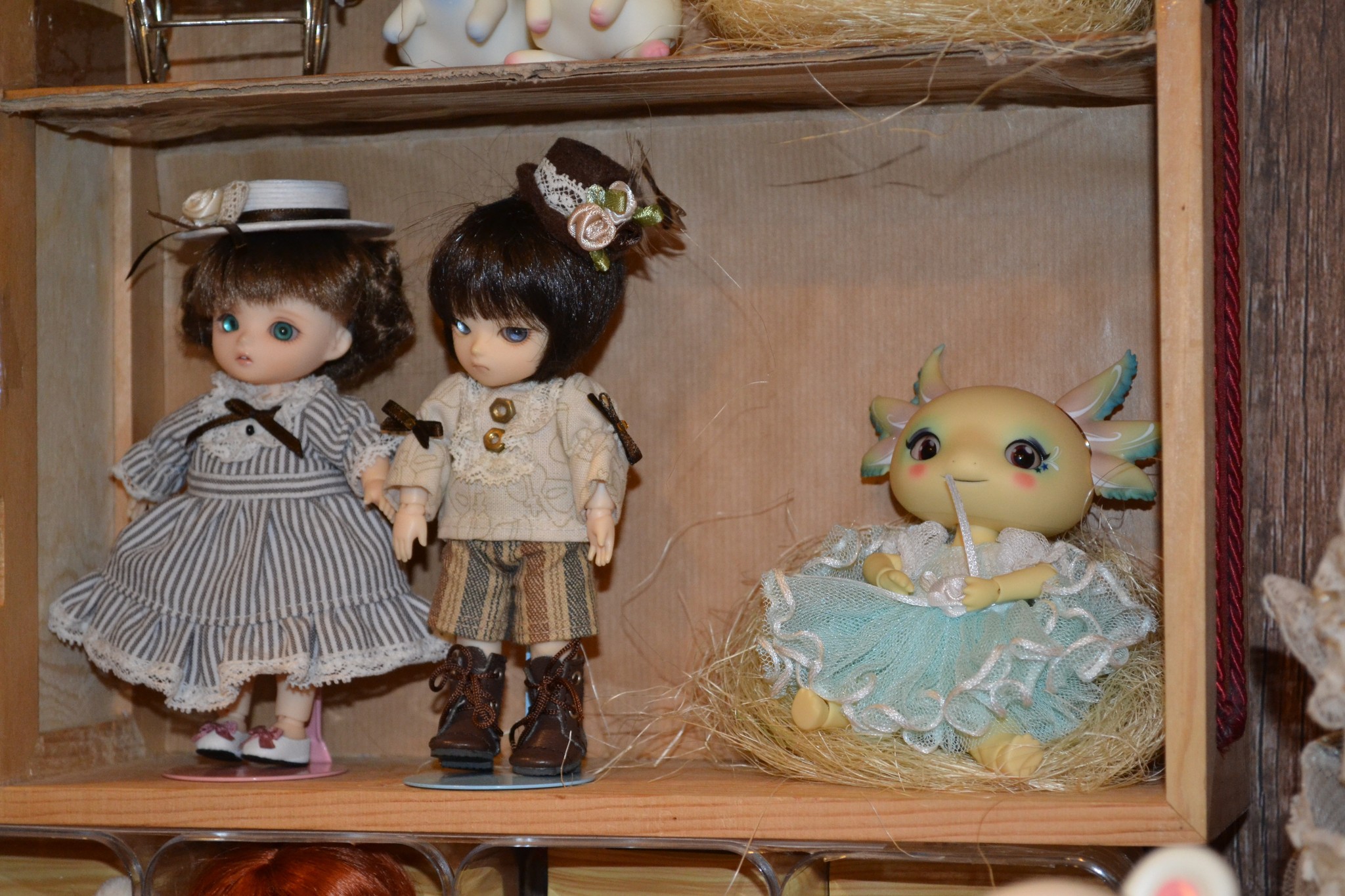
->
[45,180,453,768]
[382,140,684,775]
[758,346,1160,779]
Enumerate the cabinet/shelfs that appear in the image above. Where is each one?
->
[0,0,1261,849]
